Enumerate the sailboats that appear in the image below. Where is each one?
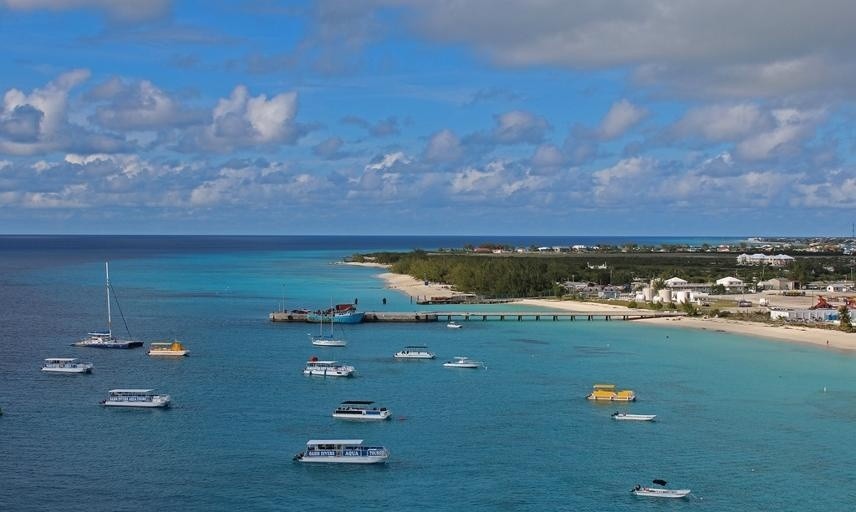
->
[306,296,350,347]
[68,261,144,350]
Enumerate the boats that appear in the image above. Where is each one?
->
[584,383,637,402]
[393,344,436,359]
[440,354,485,368]
[629,479,693,500]
[301,355,358,378]
[610,408,657,420]
[331,398,393,421]
[305,301,365,325]
[39,355,95,373]
[293,436,392,466]
[97,388,173,409]
[446,320,463,328]
[146,336,192,358]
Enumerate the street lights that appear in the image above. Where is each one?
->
[843,273,848,293]
